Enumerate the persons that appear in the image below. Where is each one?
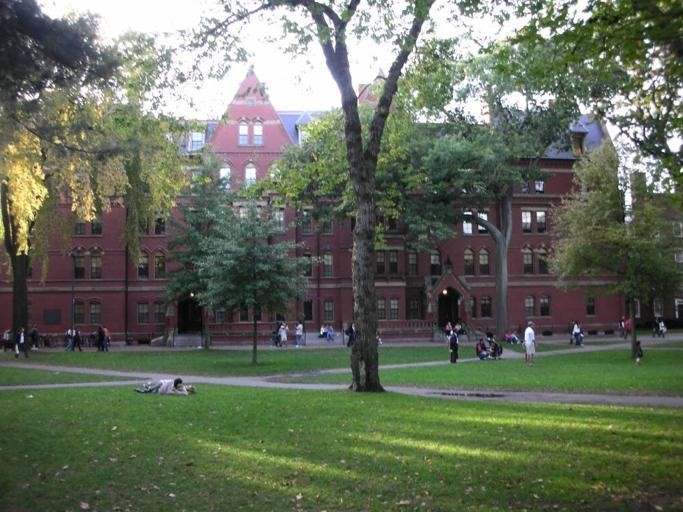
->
[445,315,667,368]
[142,378,188,395]
[319,321,354,348]
[132,379,196,393]
[272,320,303,348]
[2,324,111,357]
